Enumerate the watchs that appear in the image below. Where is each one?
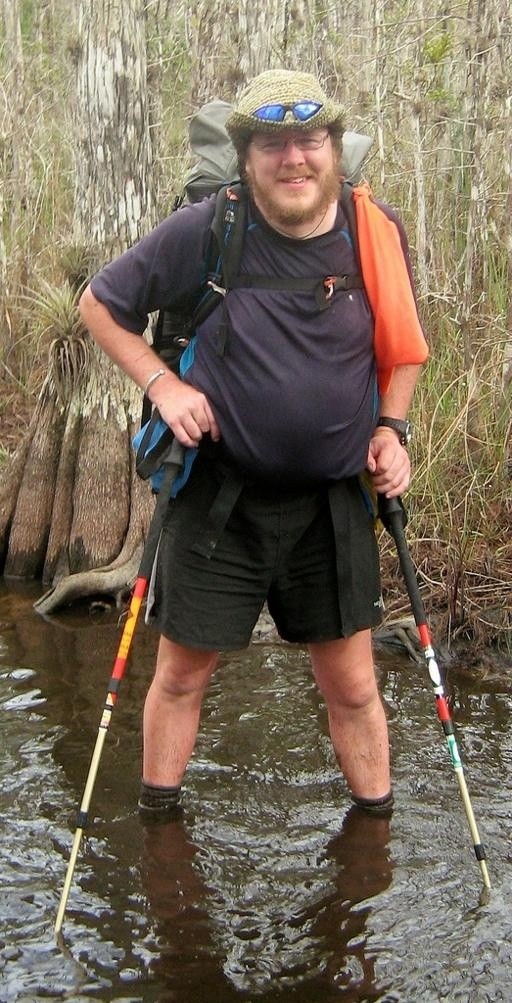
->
[375,414,415,445]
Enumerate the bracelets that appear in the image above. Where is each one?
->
[143,368,166,400]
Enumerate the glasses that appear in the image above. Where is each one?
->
[249,131,332,152]
[247,101,323,122]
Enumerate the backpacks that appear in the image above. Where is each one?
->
[148,100,381,373]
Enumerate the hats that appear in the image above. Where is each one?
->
[224,68,349,157]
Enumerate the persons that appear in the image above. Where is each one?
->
[73,68,429,820]
